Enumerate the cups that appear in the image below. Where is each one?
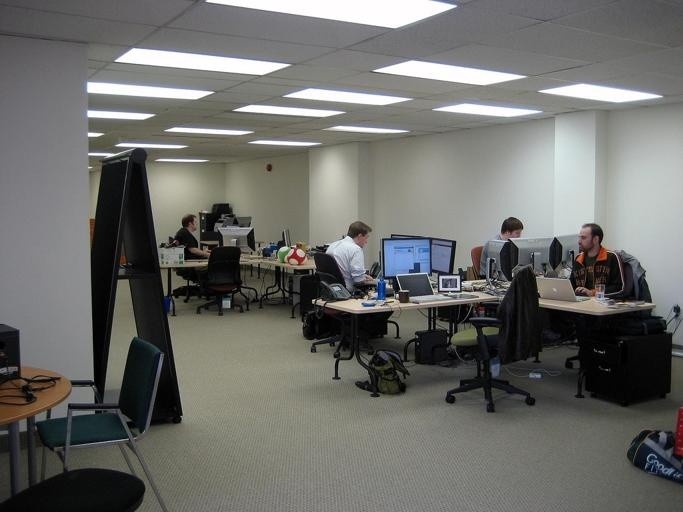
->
[595,284,604,301]
[394,290,409,303]
[203,246,208,253]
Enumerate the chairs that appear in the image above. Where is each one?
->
[36,336,169,512]
[0,467,145,512]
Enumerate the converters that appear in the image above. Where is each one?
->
[528,373,542,379]
[674,306,680,313]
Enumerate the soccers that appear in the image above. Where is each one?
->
[277,246,289,264]
[287,248,307,266]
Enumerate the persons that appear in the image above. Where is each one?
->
[174,215,211,271]
[479,217,523,279]
[325,221,373,294]
[569,224,626,300]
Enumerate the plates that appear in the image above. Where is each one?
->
[362,303,376,307]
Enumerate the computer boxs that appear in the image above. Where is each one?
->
[415,328,447,364]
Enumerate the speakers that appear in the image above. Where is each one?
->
[0,323,20,384]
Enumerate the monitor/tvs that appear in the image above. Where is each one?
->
[218,227,256,262]
[555,235,582,270]
[508,237,555,281]
[380,237,432,279]
[484,240,515,286]
[430,238,456,276]
[278,228,292,250]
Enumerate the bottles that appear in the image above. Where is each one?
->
[378,278,385,299]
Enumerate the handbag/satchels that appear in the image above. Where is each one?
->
[628,430,682,485]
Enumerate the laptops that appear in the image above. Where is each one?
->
[535,276,591,302]
[396,272,452,303]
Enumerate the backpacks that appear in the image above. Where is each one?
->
[367,350,410,394]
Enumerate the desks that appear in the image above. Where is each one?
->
[0,366,72,498]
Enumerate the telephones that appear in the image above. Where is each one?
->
[319,281,352,300]
